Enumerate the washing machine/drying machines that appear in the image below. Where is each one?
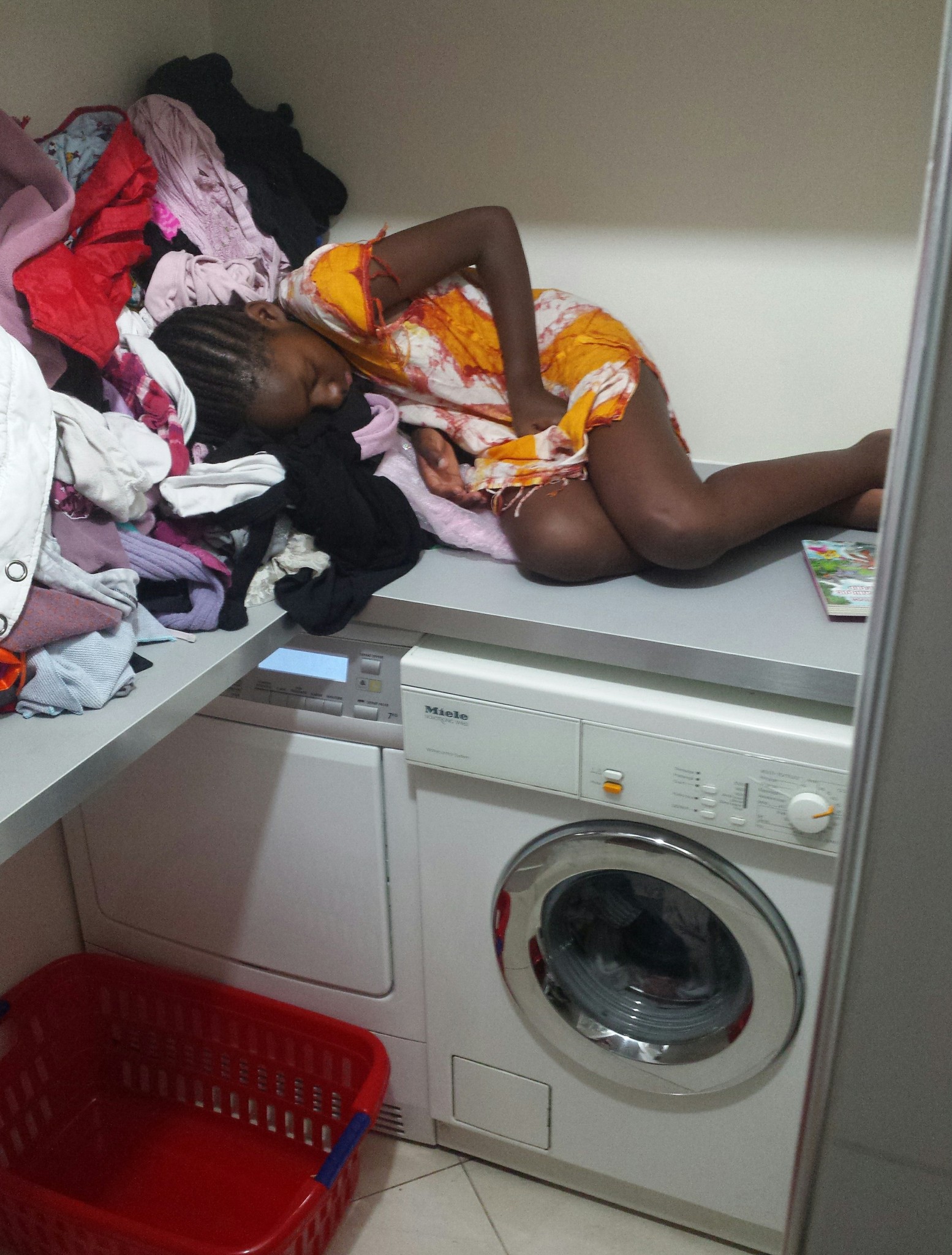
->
[397,633,854,1255]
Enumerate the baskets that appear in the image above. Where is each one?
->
[0,947,392,1255]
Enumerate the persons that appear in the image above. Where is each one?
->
[151,206,892,582]
